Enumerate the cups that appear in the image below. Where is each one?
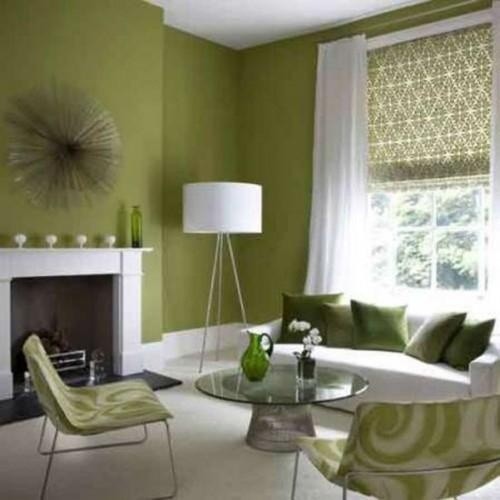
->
[294,349,318,395]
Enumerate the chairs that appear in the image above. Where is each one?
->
[22,333,178,500]
[291,392,500,500]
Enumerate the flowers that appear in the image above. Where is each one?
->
[288,317,321,364]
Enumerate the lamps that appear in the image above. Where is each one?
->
[181,181,263,373]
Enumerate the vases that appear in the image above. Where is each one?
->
[297,364,315,379]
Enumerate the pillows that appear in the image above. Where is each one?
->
[403,311,467,362]
[323,302,353,347]
[350,299,408,351]
[277,292,342,344]
[442,318,496,371]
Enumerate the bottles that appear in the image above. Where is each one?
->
[240,329,273,384]
[129,202,144,246]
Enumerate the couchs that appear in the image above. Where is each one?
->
[238,308,500,414]
